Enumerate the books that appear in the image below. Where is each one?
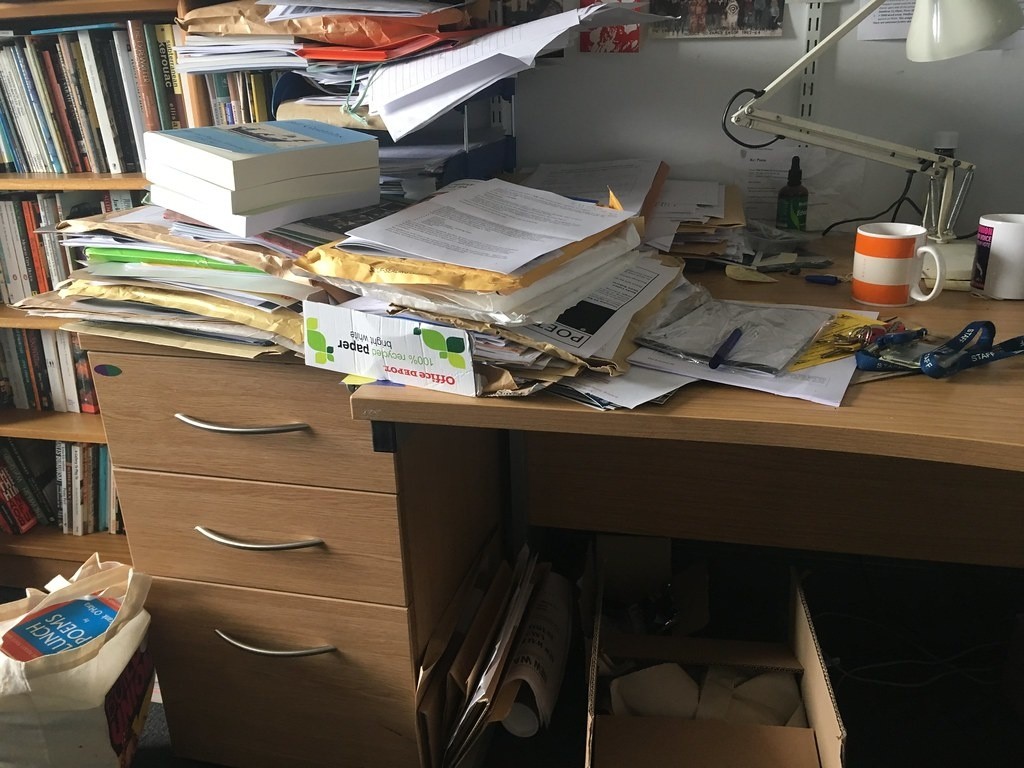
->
[626,289,880,408]
[0,0,743,768]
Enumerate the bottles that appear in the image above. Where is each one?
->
[934,132,959,181]
[776,155,808,232]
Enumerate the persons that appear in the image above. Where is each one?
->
[680,1,780,35]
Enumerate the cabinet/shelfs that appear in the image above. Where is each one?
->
[74,331,505,768]
[0,0,211,594]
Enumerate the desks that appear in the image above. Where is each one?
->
[350,230,1024,567]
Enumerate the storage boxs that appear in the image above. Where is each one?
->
[584,559,848,768]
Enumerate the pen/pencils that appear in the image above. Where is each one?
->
[708,327,744,369]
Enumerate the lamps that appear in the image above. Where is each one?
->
[721,0,1024,292]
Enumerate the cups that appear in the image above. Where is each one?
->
[850,223,947,308]
[970,213,1024,301]
